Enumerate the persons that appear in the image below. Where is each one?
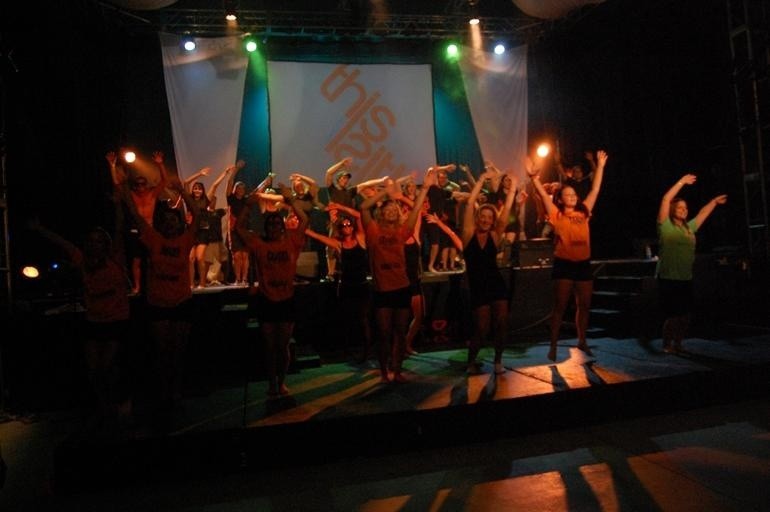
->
[655,173,727,352]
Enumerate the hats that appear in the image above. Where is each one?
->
[336,171,351,181]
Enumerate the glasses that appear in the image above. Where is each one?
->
[338,222,353,228]
